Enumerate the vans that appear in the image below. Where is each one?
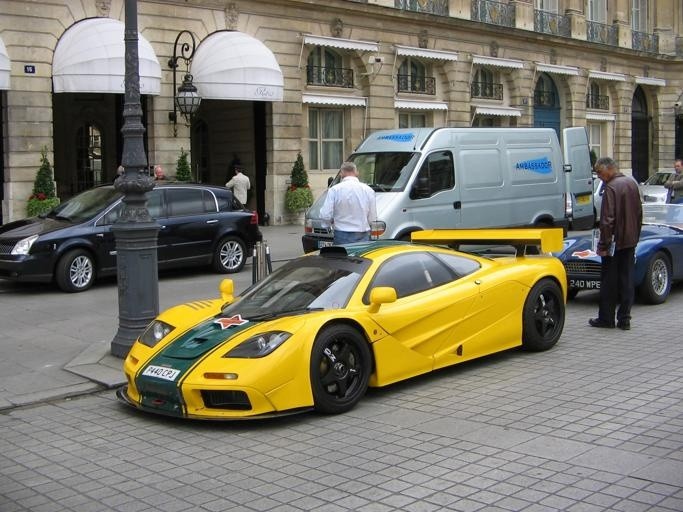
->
[296,125,598,266]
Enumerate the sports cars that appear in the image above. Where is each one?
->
[111,225,569,426]
[547,202,682,306]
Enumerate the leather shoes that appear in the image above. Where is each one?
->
[589,319,614,328]
[617,318,630,330]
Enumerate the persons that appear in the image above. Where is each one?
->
[317,160,377,246]
[223,164,252,207]
[586,155,644,331]
[662,158,682,206]
[153,165,169,181]
[110,164,123,183]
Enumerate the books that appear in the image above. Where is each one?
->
[590,230,615,257]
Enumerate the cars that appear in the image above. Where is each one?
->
[562,168,638,226]
[634,165,675,205]
[0,180,267,295]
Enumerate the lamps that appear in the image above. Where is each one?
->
[168,30,202,137]
